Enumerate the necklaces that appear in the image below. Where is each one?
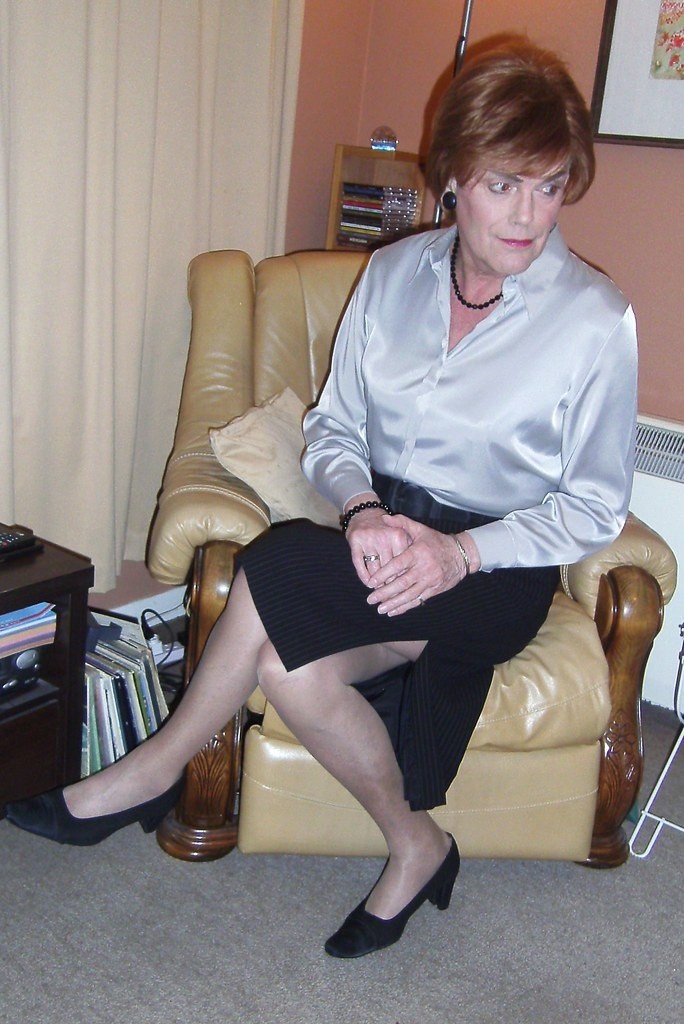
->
[451,234,504,310]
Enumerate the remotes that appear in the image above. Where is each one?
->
[0,528,33,555]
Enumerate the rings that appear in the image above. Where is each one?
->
[364,555,380,562]
[417,595,424,606]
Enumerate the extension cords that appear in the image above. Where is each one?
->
[153,640,186,667]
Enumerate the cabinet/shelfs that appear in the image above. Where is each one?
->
[0,524,95,820]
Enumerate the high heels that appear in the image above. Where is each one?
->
[324,831,461,959]
[5,755,186,846]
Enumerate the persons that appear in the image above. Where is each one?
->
[3,40,638,958]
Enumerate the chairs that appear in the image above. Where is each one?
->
[144,249,678,869]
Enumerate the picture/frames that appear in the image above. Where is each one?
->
[588,0,684,148]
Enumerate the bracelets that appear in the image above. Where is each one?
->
[449,532,470,576]
[341,501,393,535]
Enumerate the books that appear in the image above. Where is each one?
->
[0,602,56,659]
[81,604,170,780]
